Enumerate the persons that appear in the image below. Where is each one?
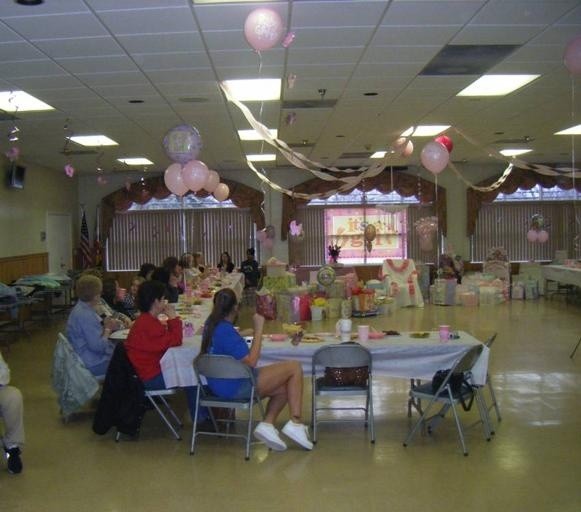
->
[0,352,26,475]
[201,287,314,452]
[217,251,234,274]
[240,248,261,289]
[65,250,224,432]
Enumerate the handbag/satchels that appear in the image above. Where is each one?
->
[324,342,368,386]
[432,369,464,396]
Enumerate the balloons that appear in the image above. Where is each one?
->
[436,133,454,154]
[202,169,220,193]
[527,228,538,243]
[244,8,284,51]
[365,225,376,242]
[531,214,544,231]
[393,135,414,161]
[162,124,202,165]
[181,160,208,192]
[213,182,231,203]
[538,230,549,244]
[255,225,276,250]
[419,141,450,177]
[564,37,581,74]
[164,163,190,196]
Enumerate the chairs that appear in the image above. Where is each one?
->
[190,354,265,461]
[311,344,376,445]
[403,345,490,457]
[115,343,184,442]
[58,332,105,424]
[427,332,502,434]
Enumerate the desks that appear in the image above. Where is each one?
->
[0,284,72,339]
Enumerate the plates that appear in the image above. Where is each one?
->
[266,330,461,344]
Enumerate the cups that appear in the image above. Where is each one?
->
[234,326,241,335]
[340,319,352,342]
[244,337,254,349]
[438,324,450,341]
[358,325,370,344]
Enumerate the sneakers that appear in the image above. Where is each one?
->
[3,444,22,474]
[281,420,313,450]
[253,422,287,451]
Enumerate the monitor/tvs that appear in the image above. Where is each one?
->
[6,164,27,190]
